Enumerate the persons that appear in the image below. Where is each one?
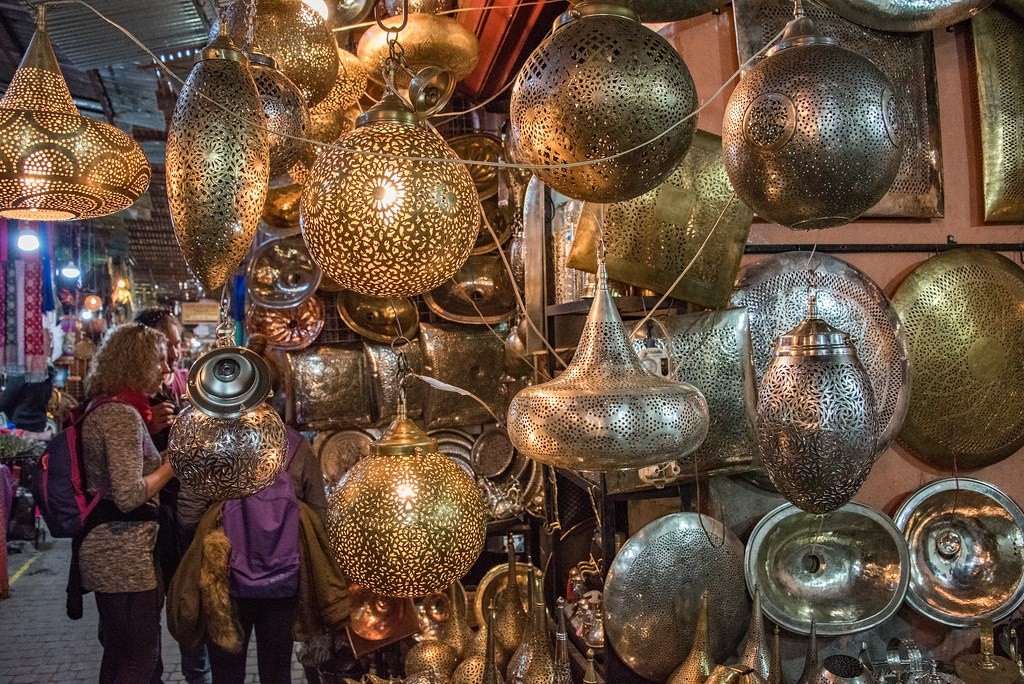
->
[71,308,322,682]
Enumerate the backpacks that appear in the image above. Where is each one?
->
[225,425,304,599]
[30,398,145,538]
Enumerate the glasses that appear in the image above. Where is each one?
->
[146,310,173,324]
[139,324,150,332]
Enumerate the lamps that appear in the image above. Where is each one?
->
[300,25,483,301]
[510,1,700,204]
[753,270,880,514]
[326,379,487,599]
[403,533,874,684]
[722,0,905,232]
[165,0,368,297]
[0,37,152,358]
[356,0,479,90]
[508,240,709,473]
[171,316,290,502]
